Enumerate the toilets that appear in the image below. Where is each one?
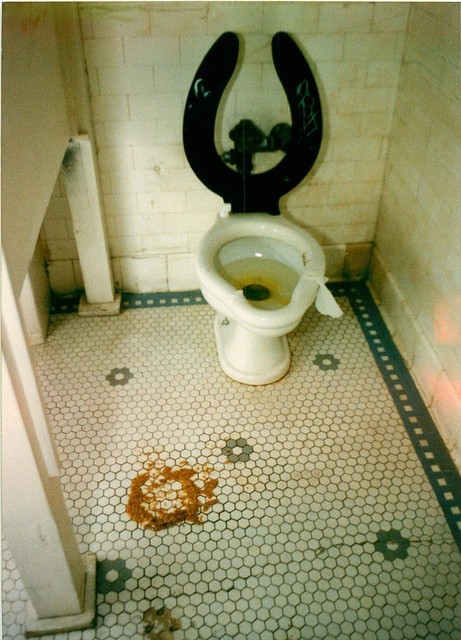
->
[187,211,327,388]
